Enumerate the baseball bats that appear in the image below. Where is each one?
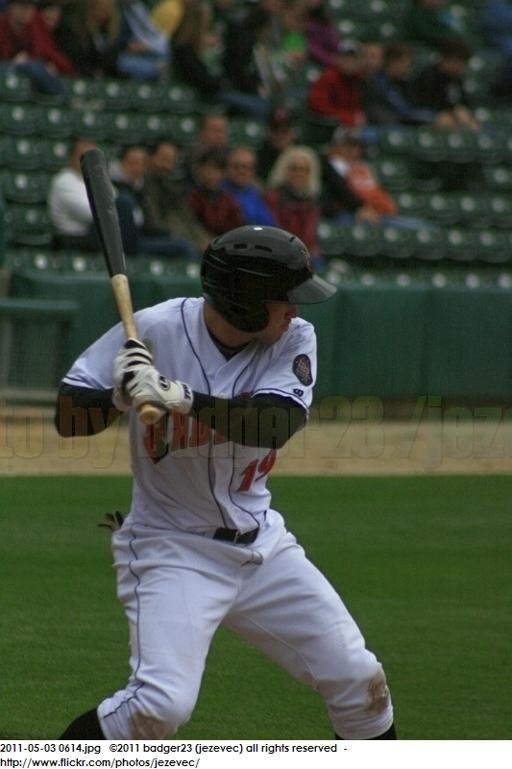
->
[79,144,164,427]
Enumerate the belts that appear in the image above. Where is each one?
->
[213,526,258,544]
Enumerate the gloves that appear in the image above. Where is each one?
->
[111,339,193,414]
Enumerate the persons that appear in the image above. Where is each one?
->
[53,226,397,740]
[0,1,511,283]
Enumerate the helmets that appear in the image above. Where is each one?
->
[200,223,338,332]
